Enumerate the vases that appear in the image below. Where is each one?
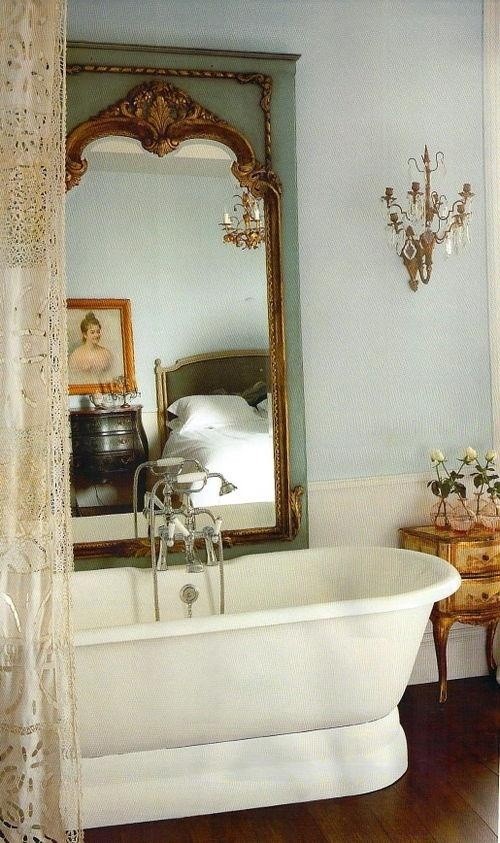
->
[429,493,500,533]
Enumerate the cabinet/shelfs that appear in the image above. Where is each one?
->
[396,516,499,710]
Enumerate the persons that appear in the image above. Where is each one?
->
[68,311,115,370]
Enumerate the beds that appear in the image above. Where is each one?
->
[148,346,276,539]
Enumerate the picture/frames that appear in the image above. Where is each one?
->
[63,294,137,396]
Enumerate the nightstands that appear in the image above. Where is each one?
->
[67,401,151,518]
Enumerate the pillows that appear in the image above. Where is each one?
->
[165,390,261,435]
[253,397,270,421]
[165,405,264,435]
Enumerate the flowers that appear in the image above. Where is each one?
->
[423,442,500,517]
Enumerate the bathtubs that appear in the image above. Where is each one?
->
[72,502,280,543]
[73,546,462,828]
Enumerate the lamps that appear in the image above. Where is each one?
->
[378,141,479,293]
[216,179,268,249]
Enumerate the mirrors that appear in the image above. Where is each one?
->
[39,36,312,571]
